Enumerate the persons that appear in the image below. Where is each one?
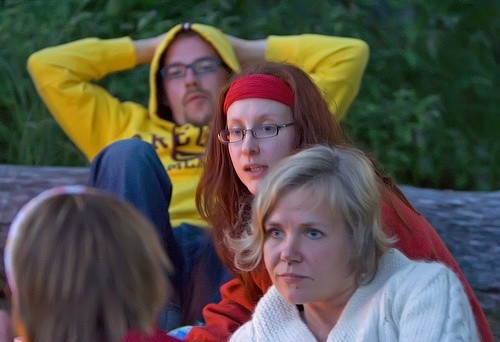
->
[25,22,372,332]
[184,61,494,342]
[1,184,181,342]
[224,144,483,342]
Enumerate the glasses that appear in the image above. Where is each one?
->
[218,122,294,144]
[161,57,221,79]
